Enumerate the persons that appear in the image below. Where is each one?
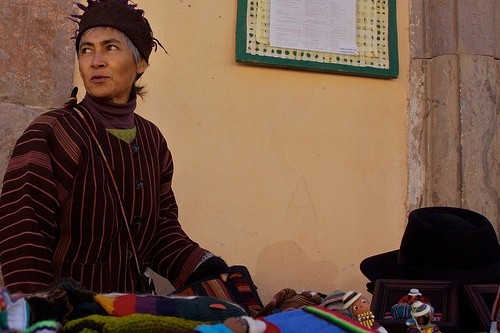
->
[0,0,229,301]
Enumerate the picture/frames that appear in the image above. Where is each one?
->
[464,283,500,333]
[370,279,458,333]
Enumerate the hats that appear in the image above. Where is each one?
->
[361,208,500,296]
[76,0,152,65]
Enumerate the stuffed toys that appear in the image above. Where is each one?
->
[194,316,281,333]
[343,291,387,333]
